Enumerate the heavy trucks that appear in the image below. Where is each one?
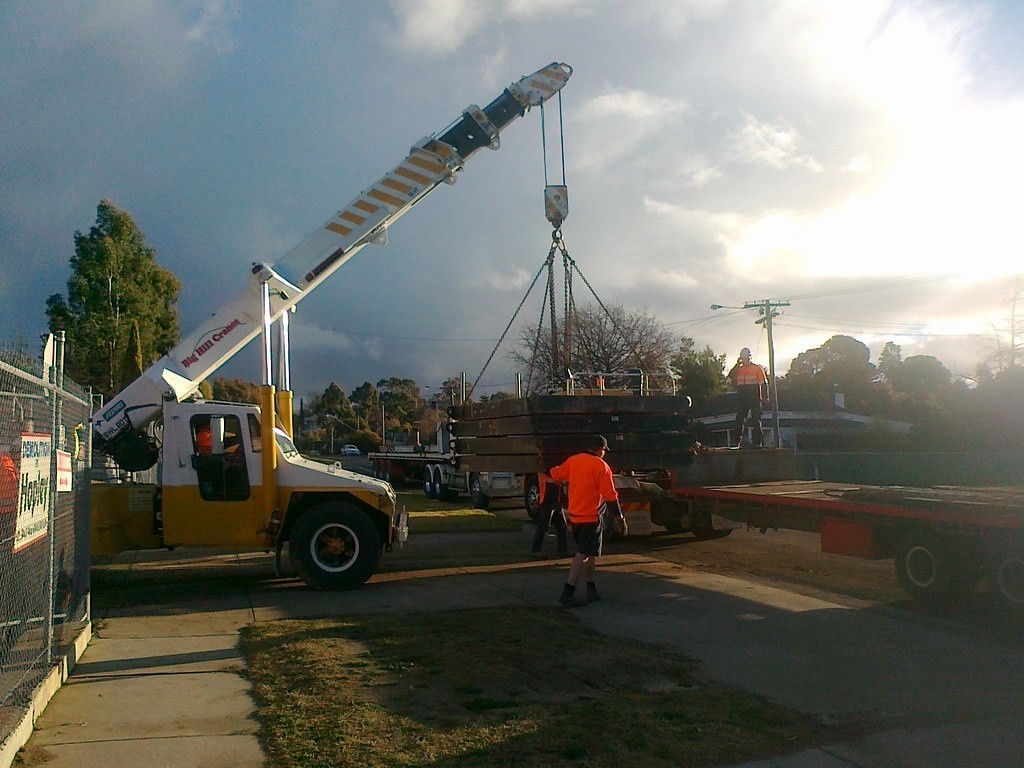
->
[365,403,529,512]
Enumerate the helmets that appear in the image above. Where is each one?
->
[740,348,751,358]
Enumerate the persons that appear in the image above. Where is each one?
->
[531,473,569,556]
[550,435,628,606]
[728,348,770,448]
[0,436,21,515]
[196,420,238,453]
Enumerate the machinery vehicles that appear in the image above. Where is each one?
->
[87,61,572,591]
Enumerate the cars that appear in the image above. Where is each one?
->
[340,445,359,457]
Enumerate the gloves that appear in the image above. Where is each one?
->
[558,495,568,512]
[614,517,628,536]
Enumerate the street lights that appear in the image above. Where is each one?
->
[711,304,781,447]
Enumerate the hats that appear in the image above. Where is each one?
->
[587,434,611,451]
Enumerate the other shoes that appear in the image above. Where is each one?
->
[586,592,600,602]
[556,547,573,558]
[729,438,741,450]
[754,439,763,448]
[560,593,582,606]
[531,550,548,561]
